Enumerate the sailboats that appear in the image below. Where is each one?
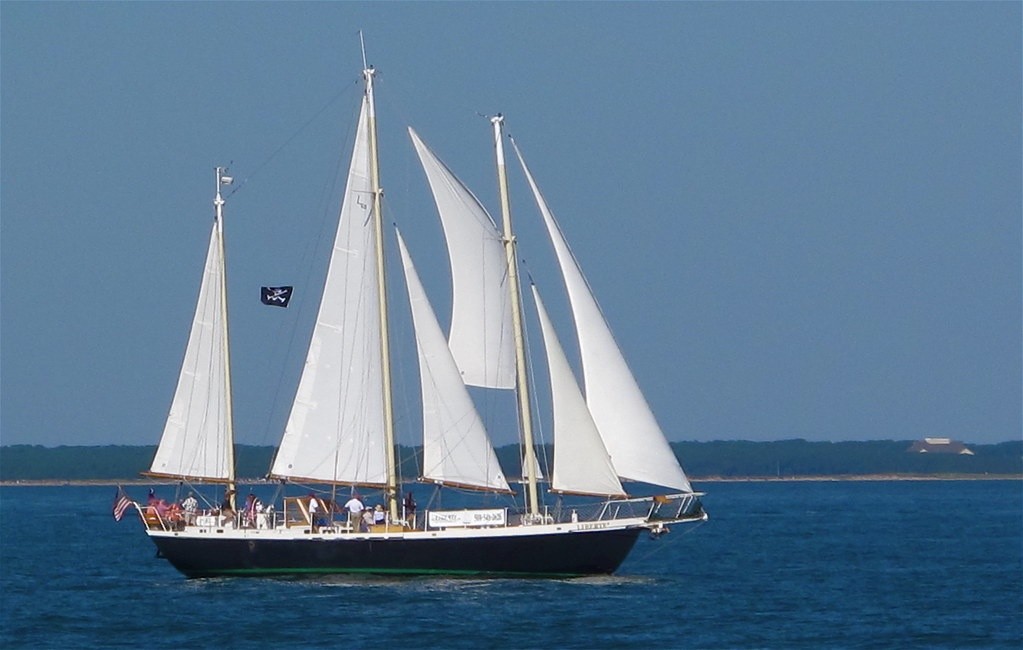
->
[134,67,708,578]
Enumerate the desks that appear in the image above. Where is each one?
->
[319,526,353,534]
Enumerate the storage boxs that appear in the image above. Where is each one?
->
[198,516,218,526]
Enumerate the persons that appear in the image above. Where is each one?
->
[340,494,385,533]
[221,490,255,528]
[146,487,198,527]
[403,492,416,529]
[309,493,318,524]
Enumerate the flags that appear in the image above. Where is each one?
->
[113,488,133,521]
[260,285,294,308]
